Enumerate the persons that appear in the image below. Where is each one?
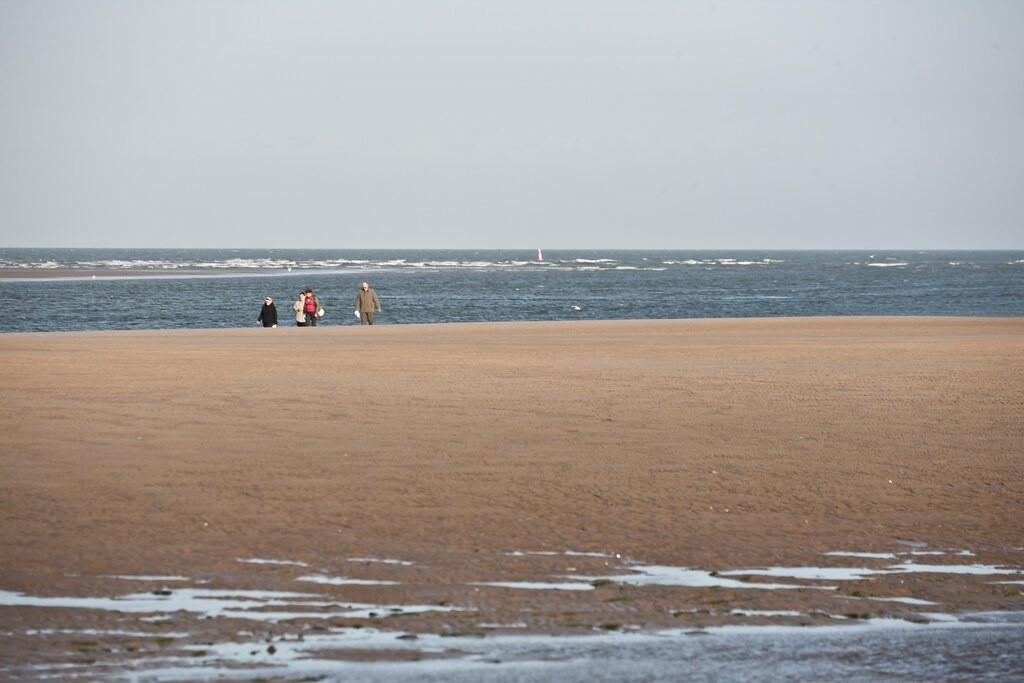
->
[293,290,320,327]
[257,297,277,328]
[355,282,382,325]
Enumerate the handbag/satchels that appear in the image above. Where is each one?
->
[317,308,325,317]
[355,310,361,318]
